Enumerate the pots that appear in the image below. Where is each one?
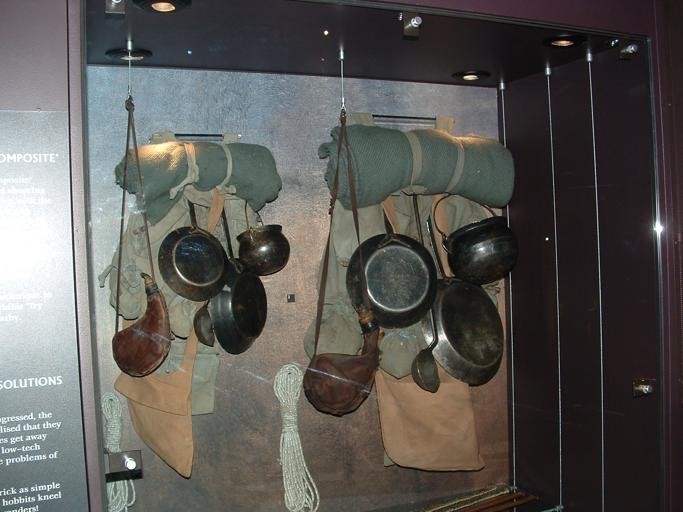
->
[345,192,516,386]
[158,192,290,355]
